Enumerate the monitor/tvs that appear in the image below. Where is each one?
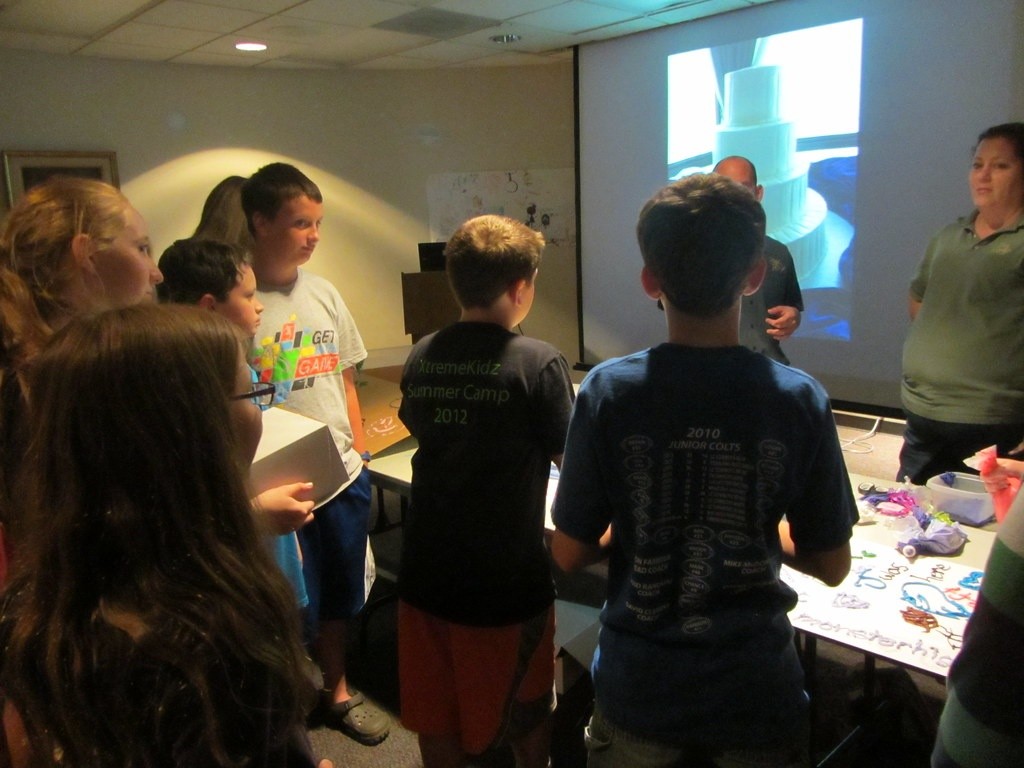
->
[418,242,449,272]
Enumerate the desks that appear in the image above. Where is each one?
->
[358,412,999,760]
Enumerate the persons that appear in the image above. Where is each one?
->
[895,121,1024,485]
[552,174,859,768]
[656,155,804,365]
[395,214,574,768]
[231,163,392,745]
[0,305,332,768]
[191,175,257,264]
[149,236,310,608]
[0,173,316,595]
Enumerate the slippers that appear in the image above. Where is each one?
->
[325,685,391,746]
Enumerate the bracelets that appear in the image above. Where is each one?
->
[360,453,371,462]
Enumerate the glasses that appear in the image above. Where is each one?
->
[231,379,277,410]
